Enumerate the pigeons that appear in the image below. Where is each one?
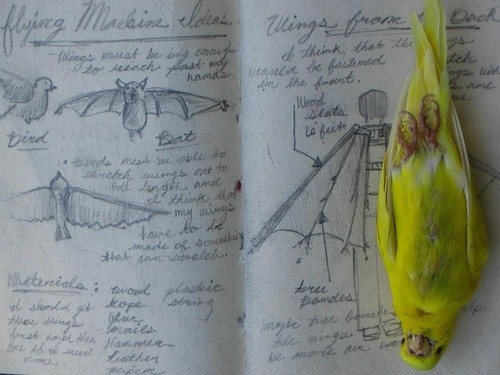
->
[0,68,57,126]
[1,169,172,241]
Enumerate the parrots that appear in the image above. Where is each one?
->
[375,0,491,371]
